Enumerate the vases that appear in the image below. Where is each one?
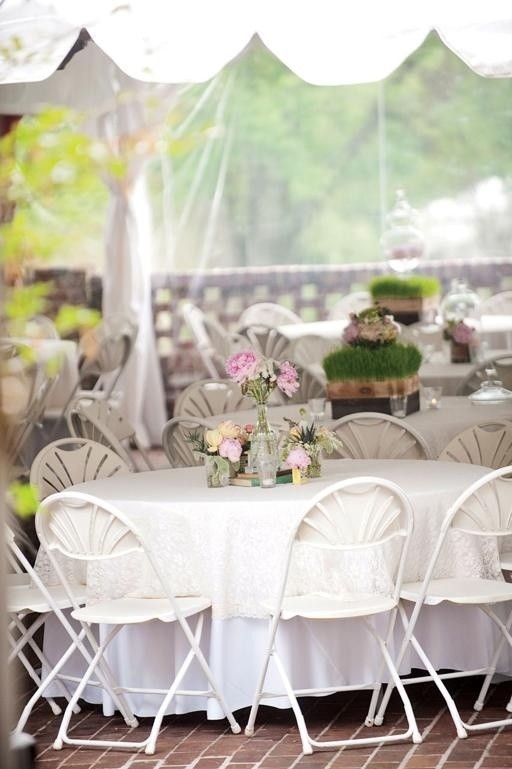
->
[254,403,279,489]
[206,455,323,486]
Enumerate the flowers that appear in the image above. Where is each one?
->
[343,310,402,344]
[201,421,341,469]
[225,353,301,397]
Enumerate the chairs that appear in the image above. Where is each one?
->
[175,303,334,394]
[311,412,430,457]
[161,417,216,467]
[6,314,134,478]
[439,424,511,464]
[175,377,256,421]
[29,438,127,498]
[373,466,512,738]
[245,477,422,756]
[3,524,134,726]
[33,493,241,757]
[439,289,509,312]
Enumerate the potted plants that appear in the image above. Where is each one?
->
[362,274,441,327]
[324,345,423,417]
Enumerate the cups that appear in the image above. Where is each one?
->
[257,453,278,487]
[390,395,406,417]
[308,398,327,423]
[422,386,442,411]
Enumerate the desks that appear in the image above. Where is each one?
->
[39,460,512,721]
